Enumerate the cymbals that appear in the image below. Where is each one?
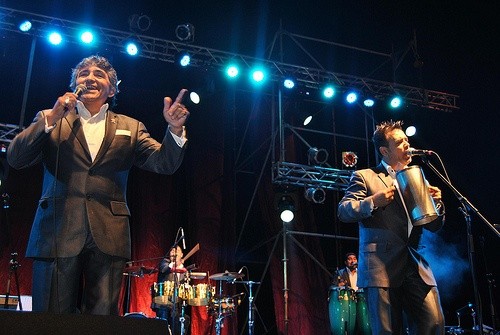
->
[124,265,159,274]
[208,272,244,280]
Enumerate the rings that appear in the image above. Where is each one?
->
[181,107,185,110]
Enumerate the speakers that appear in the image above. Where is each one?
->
[0,309,171,335]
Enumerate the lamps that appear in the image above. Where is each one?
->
[306,146,328,166]
[128,9,151,32]
[279,195,295,223]
[303,181,326,204]
[175,23,195,44]
[287,95,313,126]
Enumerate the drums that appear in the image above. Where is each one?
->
[394,164,439,226]
[327,283,374,335]
[189,284,214,306]
[149,280,179,306]
[211,297,237,318]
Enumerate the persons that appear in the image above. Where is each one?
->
[336,120,446,335]
[334,252,363,292]
[150,245,189,326]
[6,55,189,316]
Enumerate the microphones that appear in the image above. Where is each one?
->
[182,228,186,249]
[232,268,243,284]
[232,292,244,298]
[405,148,433,155]
[64,84,86,111]
